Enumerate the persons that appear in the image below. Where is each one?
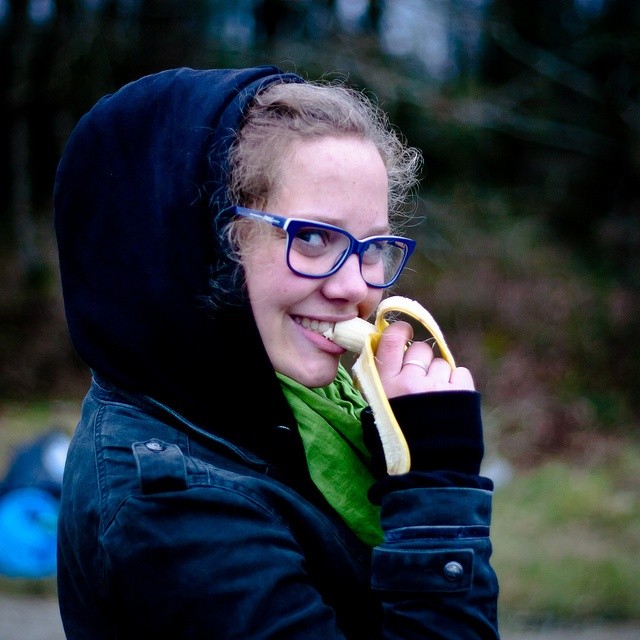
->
[57,67,498,638]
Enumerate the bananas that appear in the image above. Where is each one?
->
[324,294,457,475]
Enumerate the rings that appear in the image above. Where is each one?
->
[400,360,429,375]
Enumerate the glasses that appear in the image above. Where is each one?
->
[234,207,416,289]
